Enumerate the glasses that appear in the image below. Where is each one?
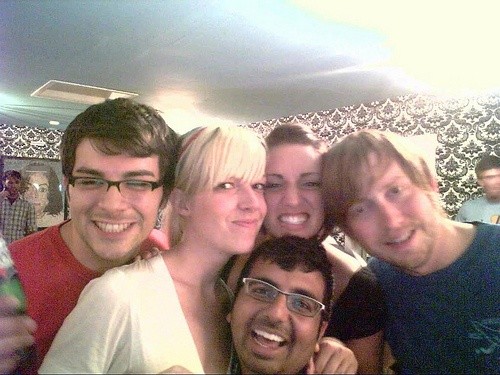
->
[238,277,330,317]
[69,173,166,199]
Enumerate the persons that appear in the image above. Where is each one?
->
[226,233,335,375]
[38,124,359,375]
[321,129,500,375]
[0,169,37,244]
[222,124,362,322]
[0,97,182,375]
[454,154,500,225]
